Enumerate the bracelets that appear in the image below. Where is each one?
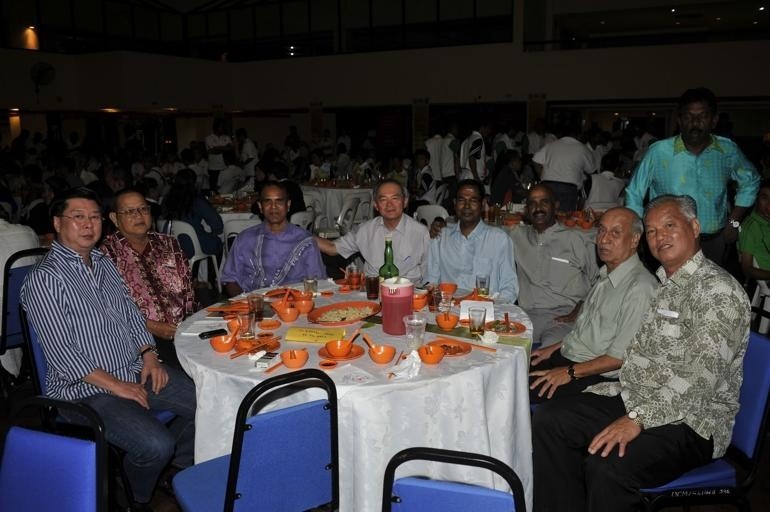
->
[141,344,157,357]
[568,361,577,388]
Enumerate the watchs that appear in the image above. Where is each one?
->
[626,410,644,430]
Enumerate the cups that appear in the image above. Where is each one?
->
[209,190,258,212]
[331,179,336,187]
[246,292,264,322]
[468,307,486,338]
[348,267,361,289]
[403,313,428,350]
[365,273,378,299]
[474,274,490,296]
[302,278,318,298]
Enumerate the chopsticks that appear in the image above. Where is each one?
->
[231,335,282,360]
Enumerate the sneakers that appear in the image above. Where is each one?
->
[158,474,172,496]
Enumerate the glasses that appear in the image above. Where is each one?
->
[117,205,151,216]
[61,214,106,224]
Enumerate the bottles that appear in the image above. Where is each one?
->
[351,162,361,188]
[377,234,399,308]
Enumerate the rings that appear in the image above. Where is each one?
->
[169,335,173,341]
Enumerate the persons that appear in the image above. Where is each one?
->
[627,88,762,262]
[525,207,659,403]
[218,179,326,299]
[97,189,203,368]
[632,121,659,162]
[429,185,597,346]
[19,191,196,506]
[419,179,520,305]
[1,120,259,272]
[259,117,626,232]
[532,192,750,509]
[1,214,41,394]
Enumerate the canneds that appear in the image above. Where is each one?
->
[366,274,379,300]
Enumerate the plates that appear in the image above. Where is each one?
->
[317,345,365,360]
[319,360,337,368]
[454,297,495,307]
[235,339,281,353]
[320,292,333,297]
[339,286,352,294]
[335,279,365,289]
[209,299,247,332]
[484,319,526,337]
[257,321,282,330]
[256,332,273,338]
[307,301,381,325]
[430,338,473,359]
[266,289,303,302]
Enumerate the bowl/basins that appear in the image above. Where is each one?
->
[485,200,606,229]
[279,350,308,367]
[212,335,235,354]
[367,345,397,364]
[439,283,458,294]
[413,294,428,311]
[278,308,300,322]
[293,300,315,314]
[270,300,293,315]
[326,340,352,356]
[419,346,445,363]
[437,315,458,332]
